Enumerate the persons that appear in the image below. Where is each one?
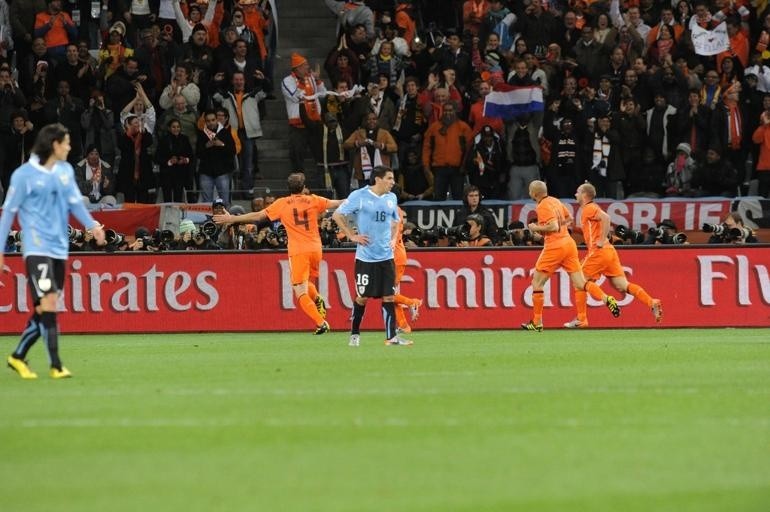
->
[563,183,664,328]
[0,122,106,379]
[522,180,621,333]
[1,2,769,253]
[394,207,423,333]
[332,167,415,347]
[213,173,347,336]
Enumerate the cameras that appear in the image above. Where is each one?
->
[267,232,280,239]
[143,236,151,243]
[368,139,373,144]
[647,227,668,240]
[192,231,205,243]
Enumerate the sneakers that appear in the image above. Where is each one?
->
[7,354,38,380]
[521,320,543,333]
[564,318,589,329]
[49,366,72,379]
[315,295,327,319]
[605,295,620,318]
[313,320,330,335]
[349,333,361,347]
[648,298,663,323]
[383,298,426,347]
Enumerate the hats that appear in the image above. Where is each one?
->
[213,198,227,211]
[180,218,196,236]
[288,51,311,70]
[229,204,246,215]
[657,219,676,229]
